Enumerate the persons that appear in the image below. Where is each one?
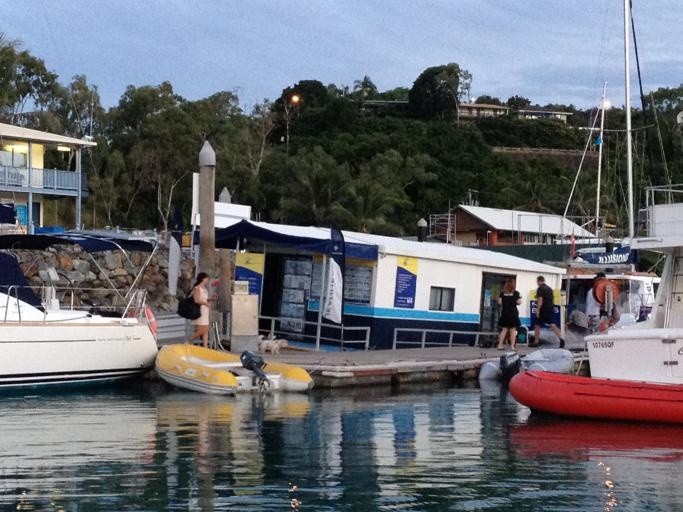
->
[527,276,565,348]
[186,272,209,349]
[497,279,521,350]
[585,272,605,336]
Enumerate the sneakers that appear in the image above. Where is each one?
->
[529,342,538,346]
[560,338,564,348]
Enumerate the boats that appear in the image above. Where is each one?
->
[160,344,315,396]
[0,231,158,391]
[509,183,682,426]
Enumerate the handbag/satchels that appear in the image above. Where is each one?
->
[178,299,201,319]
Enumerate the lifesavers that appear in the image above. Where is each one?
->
[129,308,157,341]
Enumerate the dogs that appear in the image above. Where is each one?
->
[258,334,289,357]
